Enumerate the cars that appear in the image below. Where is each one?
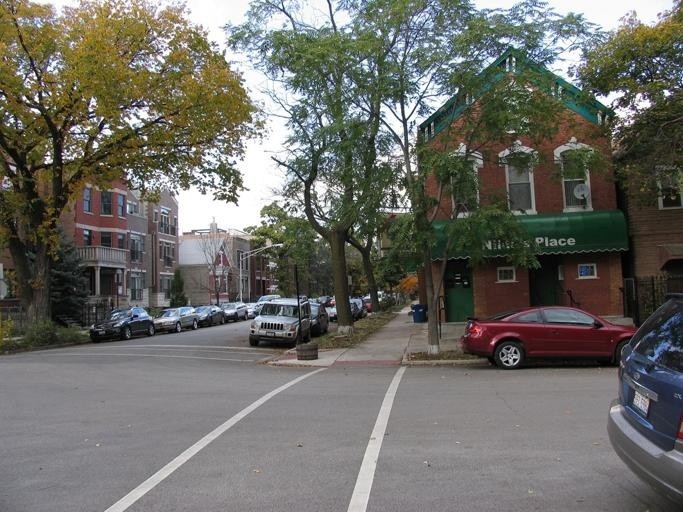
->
[297,294,396,339]
[461,304,638,371]
[150,301,249,334]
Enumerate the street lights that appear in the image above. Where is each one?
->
[238,243,285,302]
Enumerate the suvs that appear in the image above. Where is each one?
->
[88,305,156,342]
[604,290,683,504]
[248,297,313,347]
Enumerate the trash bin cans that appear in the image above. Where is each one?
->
[410,304,428,323]
